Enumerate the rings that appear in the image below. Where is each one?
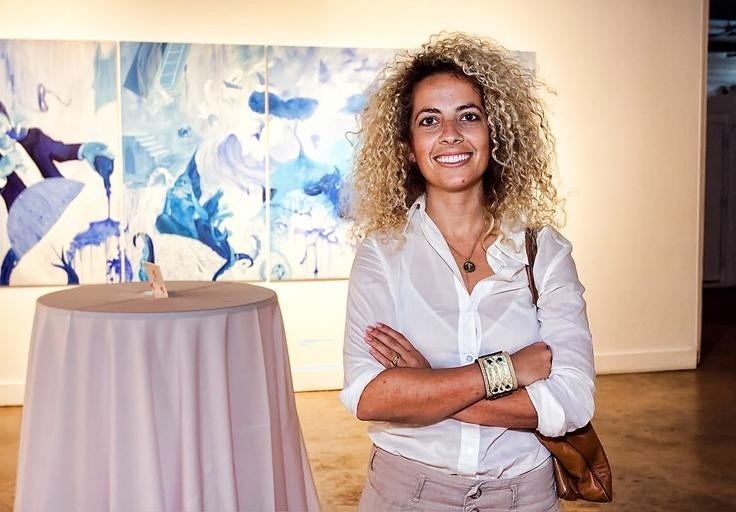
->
[391,354,399,367]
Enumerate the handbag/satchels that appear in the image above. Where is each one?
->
[533,422,612,503]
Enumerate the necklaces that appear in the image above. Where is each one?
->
[447,224,487,274]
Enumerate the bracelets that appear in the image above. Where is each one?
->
[476,350,519,401]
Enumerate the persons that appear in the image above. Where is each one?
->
[0,100,114,286]
[338,30,597,512]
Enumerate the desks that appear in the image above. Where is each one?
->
[12,279,323,512]
